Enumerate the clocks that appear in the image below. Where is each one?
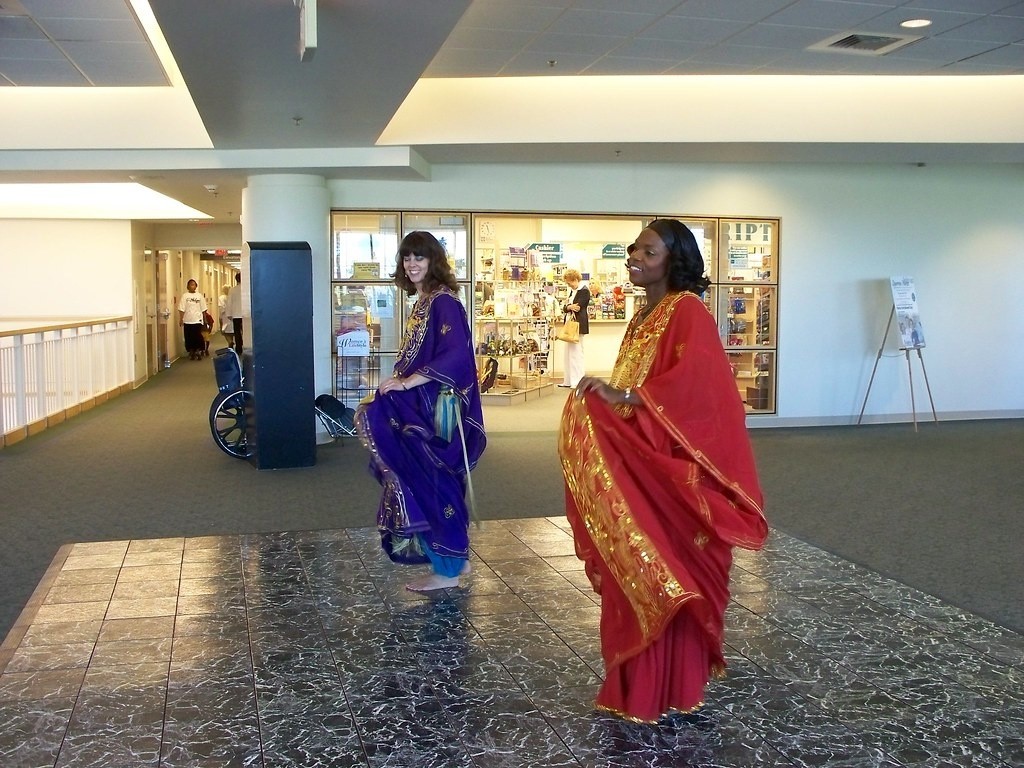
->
[479,220,496,239]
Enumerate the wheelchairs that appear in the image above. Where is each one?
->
[210,347,255,460]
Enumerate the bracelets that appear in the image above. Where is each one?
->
[399,378,408,391]
[624,385,631,403]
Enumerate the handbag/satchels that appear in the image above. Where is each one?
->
[557,310,580,343]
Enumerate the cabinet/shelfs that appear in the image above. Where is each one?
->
[477,280,553,406]
[553,263,572,308]
[624,266,770,410]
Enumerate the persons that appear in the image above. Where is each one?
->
[217,284,235,349]
[534,281,560,370]
[226,272,243,355]
[558,265,590,389]
[350,231,488,591]
[901,316,920,346]
[178,279,214,361]
[558,219,771,723]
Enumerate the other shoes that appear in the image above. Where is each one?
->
[201,352,204,357]
[190,354,195,360]
[205,350,209,356]
[558,384,571,387]
[196,354,202,360]
[229,342,234,348]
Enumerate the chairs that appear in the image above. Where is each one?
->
[314,394,358,445]
